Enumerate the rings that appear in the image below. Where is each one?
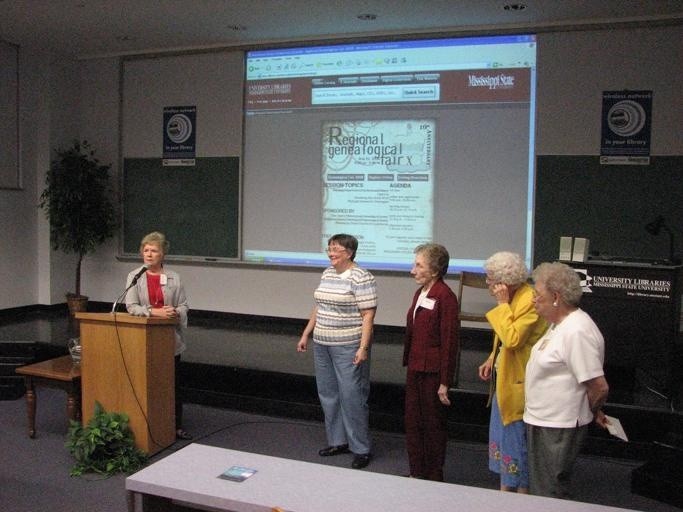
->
[440,398,444,401]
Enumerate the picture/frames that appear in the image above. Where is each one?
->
[599,90,652,166]
[163,105,196,167]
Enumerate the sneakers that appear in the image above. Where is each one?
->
[176,427,192,439]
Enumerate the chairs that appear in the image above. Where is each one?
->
[451,270,491,390]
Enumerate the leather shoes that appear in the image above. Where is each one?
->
[319,444,349,457]
[352,453,369,469]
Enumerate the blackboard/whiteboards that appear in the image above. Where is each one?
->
[114,25,683,283]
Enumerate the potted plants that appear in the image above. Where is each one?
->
[37,137,124,316]
[62,399,144,483]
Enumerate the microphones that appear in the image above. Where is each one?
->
[132,263,151,287]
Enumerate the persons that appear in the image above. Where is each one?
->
[523,262,612,500]
[402,242,460,482]
[125,231,193,440]
[360,346,369,350]
[297,234,379,469]
[478,250,551,495]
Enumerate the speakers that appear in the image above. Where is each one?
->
[572,238,589,263]
[559,237,572,260]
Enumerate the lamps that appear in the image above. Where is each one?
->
[503,4,527,12]
[357,14,377,21]
[645,213,675,261]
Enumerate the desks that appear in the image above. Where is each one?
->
[554,256,682,408]
[125,442,649,512]
[14,354,81,438]
[72,310,180,459]
